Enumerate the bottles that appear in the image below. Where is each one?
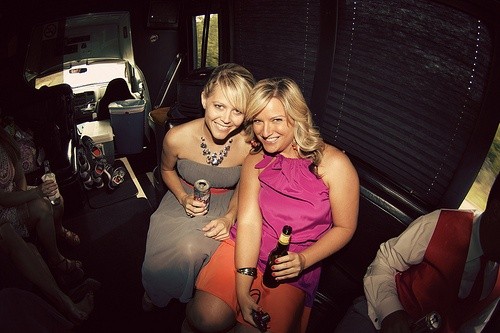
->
[43,160,60,205]
[263,225,292,288]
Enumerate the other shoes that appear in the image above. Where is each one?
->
[59,227,80,244]
[52,258,81,273]
[142,292,154,312]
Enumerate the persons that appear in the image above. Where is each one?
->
[0,113,89,326]
[141,62,260,333]
[337,174,500,333]
[179,76,360,333]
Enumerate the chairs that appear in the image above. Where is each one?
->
[97,78,134,121]
[0,84,85,215]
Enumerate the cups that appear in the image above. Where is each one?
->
[41,172,60,201]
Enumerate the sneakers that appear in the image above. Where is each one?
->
[77,136,126,190]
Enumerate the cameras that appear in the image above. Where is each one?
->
[252,309,271,332]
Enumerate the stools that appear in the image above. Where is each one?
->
[148,107,170,188]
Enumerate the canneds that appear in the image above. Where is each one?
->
[409,311,441,333]
[194,179,209,211]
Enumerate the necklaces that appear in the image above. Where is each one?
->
[200,136,233,165]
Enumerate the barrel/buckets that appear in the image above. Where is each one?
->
[108,98,147,154]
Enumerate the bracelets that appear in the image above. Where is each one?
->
[237,267,258,276]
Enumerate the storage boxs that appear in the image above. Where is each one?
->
[76,120,115,167]
